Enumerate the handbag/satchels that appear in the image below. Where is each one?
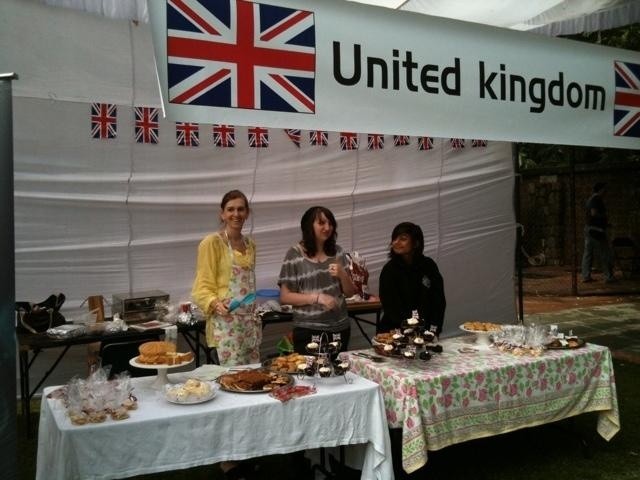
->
[15,293,67,336]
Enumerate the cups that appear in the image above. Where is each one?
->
[383,318,438,362]
[298,338,352,378]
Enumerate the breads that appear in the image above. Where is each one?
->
[272,353,309,374]
[215,366,290,391]
[463,322,503,332]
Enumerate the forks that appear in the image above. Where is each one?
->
[352,351,383,363]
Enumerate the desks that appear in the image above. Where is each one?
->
[338,330,610,474]
[15,301,384,426]
[42,362,383,480]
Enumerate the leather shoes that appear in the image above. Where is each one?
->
[215,462,244,480]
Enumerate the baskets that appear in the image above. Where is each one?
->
[373,337,403,359]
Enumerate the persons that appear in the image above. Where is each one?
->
[277,207,354,354]
[375,222,446,340]
[190,191,264,366]
[582,181,622,286]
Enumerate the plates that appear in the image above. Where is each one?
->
[542,334,584,352]
[217,369,293,393]
[167,383,219,404]
[188,361,230,382]
[265,353,313,377]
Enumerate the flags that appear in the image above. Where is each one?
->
[340,132,358,149]
[418,137,433,149]
[451,138,485,148]
[213,124,236,147]
[167,0,315,114]
[310,130,328,146]
[286,129,301,148]
[92,103,118,138]
[394,135,409,146]
[135,106,159,144]
[176,122,200,146]
[367,134,384,149]
[613,60,640,137]
[248,126,269,147]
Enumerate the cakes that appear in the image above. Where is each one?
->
[547,334,579,348]
[303,341,349,378]
[168,378,211,403]
[372,310,444,363]
[137,341,194,366]
